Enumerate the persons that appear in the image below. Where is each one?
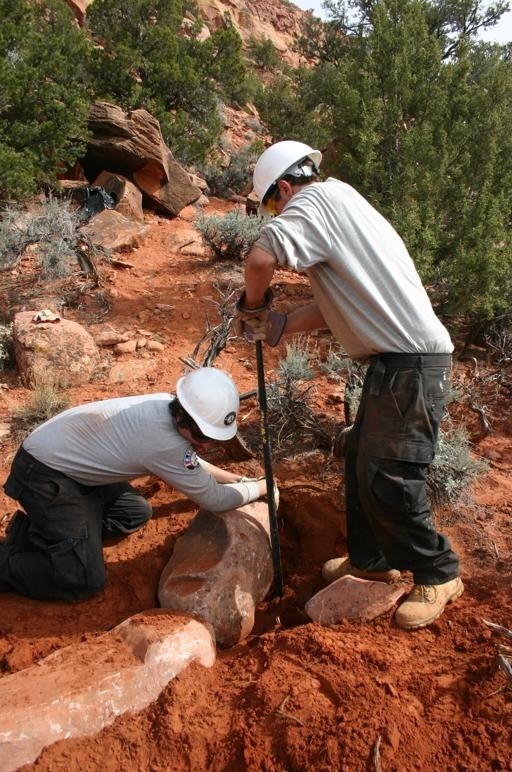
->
[0,367,279,603]
[238,141,465,629]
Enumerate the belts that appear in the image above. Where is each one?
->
[370,350,455,371]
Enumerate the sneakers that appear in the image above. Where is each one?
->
[395,574,466,631]
[323,556,402,585]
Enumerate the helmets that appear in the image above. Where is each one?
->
[251,141,324,218]
[175,367,240,441]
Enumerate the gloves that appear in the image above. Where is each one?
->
[239,475,281,513]
[229,310,287,348]
[228,286,273,346]
